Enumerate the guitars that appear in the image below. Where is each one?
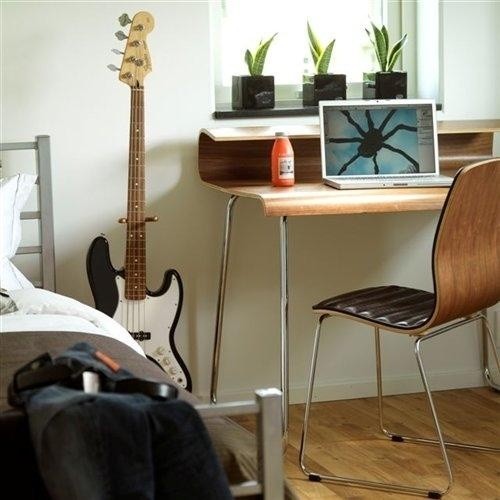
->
[86,12,191,392]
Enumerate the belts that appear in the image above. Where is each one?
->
[7,365,179,408]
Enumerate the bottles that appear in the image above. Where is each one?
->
[271,133,295,188]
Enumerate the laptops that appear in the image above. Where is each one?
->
[318,98,455,190]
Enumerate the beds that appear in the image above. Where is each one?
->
[1,132,292,500]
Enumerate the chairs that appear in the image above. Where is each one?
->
[297,157,500,494]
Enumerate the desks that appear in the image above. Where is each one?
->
[192,118,499,456]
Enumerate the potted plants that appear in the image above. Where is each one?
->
[231,17,411,111]
